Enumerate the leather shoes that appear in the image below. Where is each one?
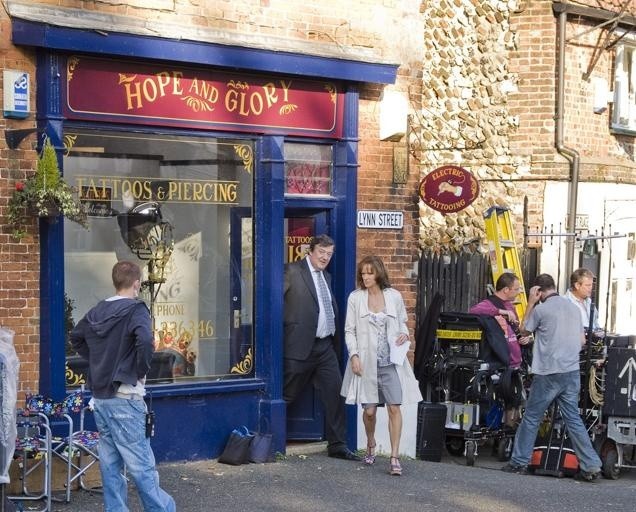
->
[328,448,362,461]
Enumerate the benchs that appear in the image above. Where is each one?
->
[65,351,176,388]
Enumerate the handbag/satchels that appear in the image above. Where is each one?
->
[218,424,251,466]
[251,414,273,462]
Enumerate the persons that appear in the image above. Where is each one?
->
[71,260,176,512]
[502,274,603,483]
[338,254,424,475]
[282,233,365,462]
[469,271,535,427]
[562,267,601,332]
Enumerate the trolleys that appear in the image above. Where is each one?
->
[426,312,636,480]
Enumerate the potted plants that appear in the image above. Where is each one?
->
[5,138,90,240]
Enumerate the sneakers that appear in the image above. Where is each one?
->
[500,460,528,475]
[574,468,600,482]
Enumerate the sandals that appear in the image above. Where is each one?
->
[365,440,376,464]
[389,456,402,475]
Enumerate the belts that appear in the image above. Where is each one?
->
[114,392,143,401]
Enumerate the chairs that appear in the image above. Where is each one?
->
[5,389,100,512]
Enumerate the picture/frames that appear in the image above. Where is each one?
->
[78,186,113,218]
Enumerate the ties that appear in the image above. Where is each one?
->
[317,271,336,337]
